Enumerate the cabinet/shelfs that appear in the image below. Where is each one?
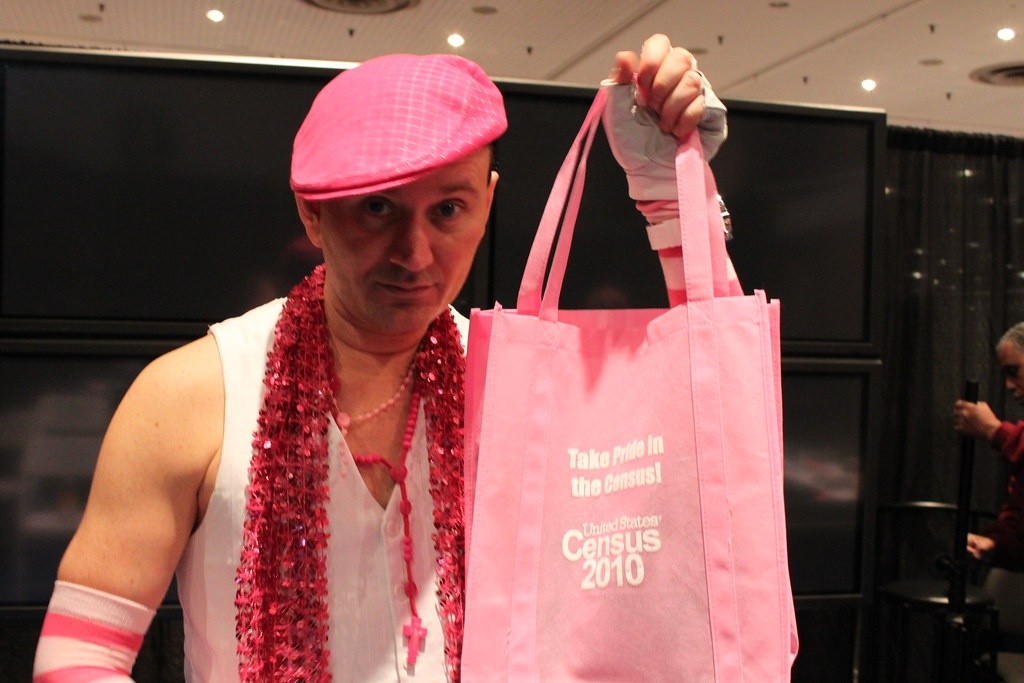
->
[0,45,890,682]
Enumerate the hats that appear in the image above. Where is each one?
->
[293,55,507,201]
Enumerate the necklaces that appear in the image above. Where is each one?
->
[316,358,426,669]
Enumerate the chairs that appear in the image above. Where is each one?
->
[873,500,1001,683]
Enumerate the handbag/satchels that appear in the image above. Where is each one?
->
[461,71,799,683]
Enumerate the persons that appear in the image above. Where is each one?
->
[952,320,1023,571]
[30,33,745,683]
